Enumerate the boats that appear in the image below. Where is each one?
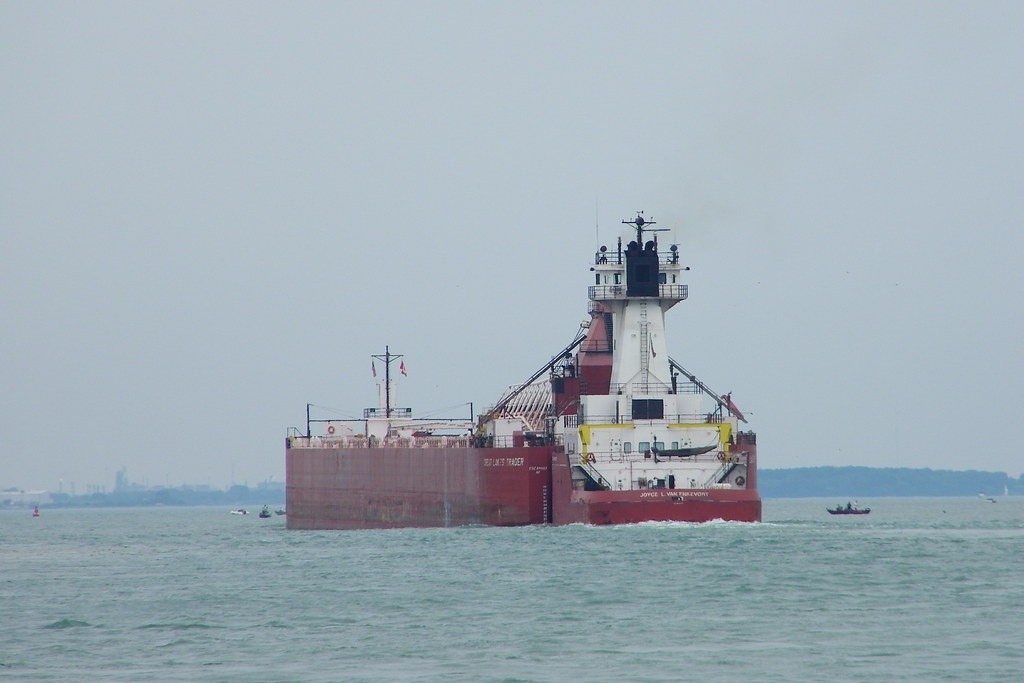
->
[275,510,286,516]
[230,509,249,515]
[259,512,271,518]
[825,507,870,515]
[657,444,718,457]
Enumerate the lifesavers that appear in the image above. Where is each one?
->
[716,451,725,461]
[735,475,745,486]
[586,453,595,461]
[328,426,335,433]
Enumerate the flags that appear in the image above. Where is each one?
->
[400,361,407,377]
[372,357,377,377]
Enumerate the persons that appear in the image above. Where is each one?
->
[33,506,38,515]
[854,500,859,510]
[847,501,852,511]
[836,504,843,511]
[471,433,493,448]
[395,434,402,447]
[545,418,555,435]
[262,505,270,513]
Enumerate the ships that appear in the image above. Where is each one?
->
[280,205,765,536]
